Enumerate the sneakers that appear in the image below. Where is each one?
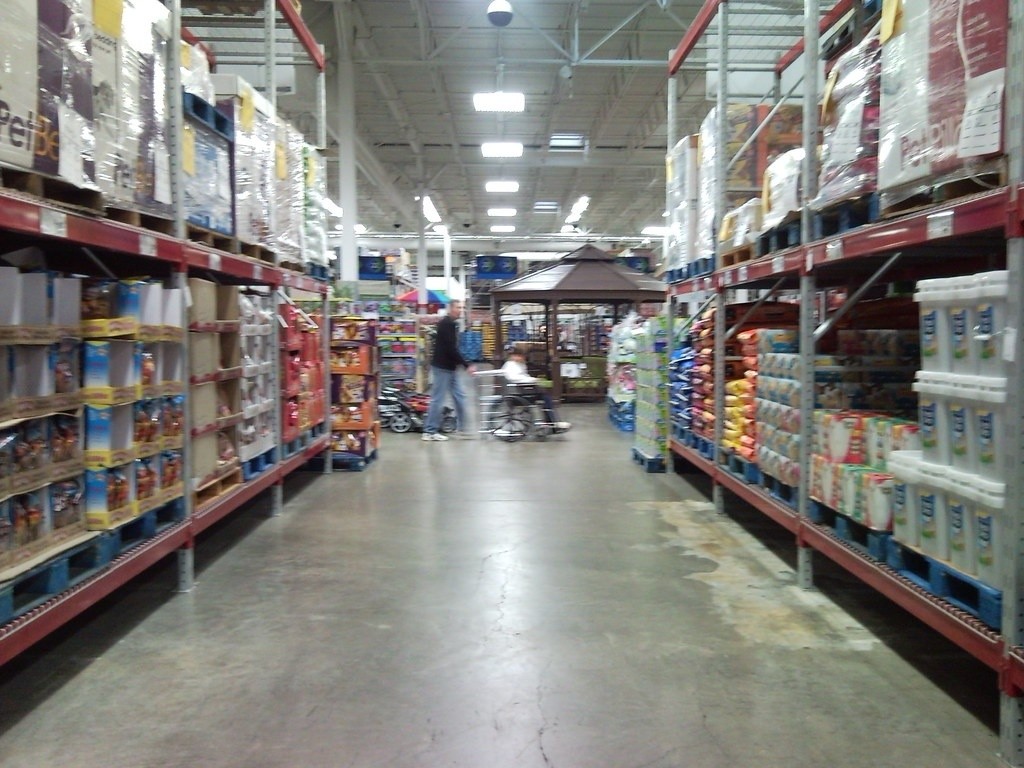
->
[421,430,449,442]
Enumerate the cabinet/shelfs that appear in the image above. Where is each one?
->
[501,316,614,357]
[664,0,1024,768]
[377,313,429,395]
[310,314,381,471]
[0,0,335,665]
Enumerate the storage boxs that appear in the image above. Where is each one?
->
[665,104,824,271]
[279,304,305,444]
[85,317,186,531]
[212,74,274,123]
[187,278,239,481]
[332,317,381,457]
[885,270,1009,590]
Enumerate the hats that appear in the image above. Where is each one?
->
[508,346,522,355]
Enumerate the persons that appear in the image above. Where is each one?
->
[420,298,478,442]
[502,347,571,433]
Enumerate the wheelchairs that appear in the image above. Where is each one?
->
[469,367,571,442]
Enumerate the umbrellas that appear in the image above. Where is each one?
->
[397,289,452,305]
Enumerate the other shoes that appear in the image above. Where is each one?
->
[554,421,573,432]
